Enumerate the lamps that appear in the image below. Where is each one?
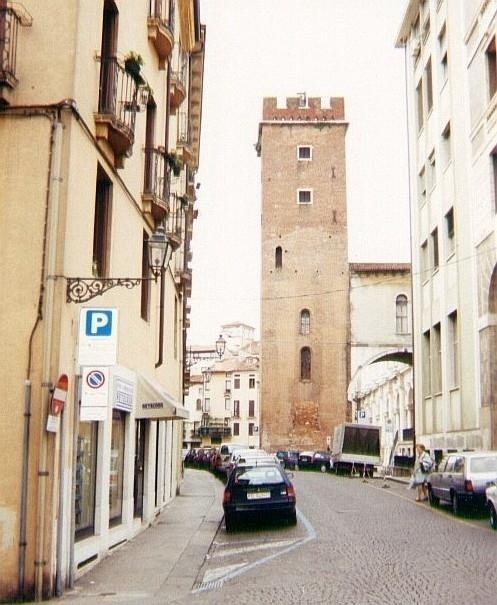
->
[55,217,172,303]
[185,334,226,360]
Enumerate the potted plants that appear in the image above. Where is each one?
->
[122,50,144,78]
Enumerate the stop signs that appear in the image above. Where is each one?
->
[50,373,69,417]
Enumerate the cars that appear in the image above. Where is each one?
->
[485,484,497,529]
[184,444,329,526]
[425,450,497,513]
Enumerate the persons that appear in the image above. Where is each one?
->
[410,444,434,502]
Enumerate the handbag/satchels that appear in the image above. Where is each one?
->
[420,451,435,474]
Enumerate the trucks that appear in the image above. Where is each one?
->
[327,424,384,479]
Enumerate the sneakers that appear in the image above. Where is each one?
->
[415,495,429,503]
